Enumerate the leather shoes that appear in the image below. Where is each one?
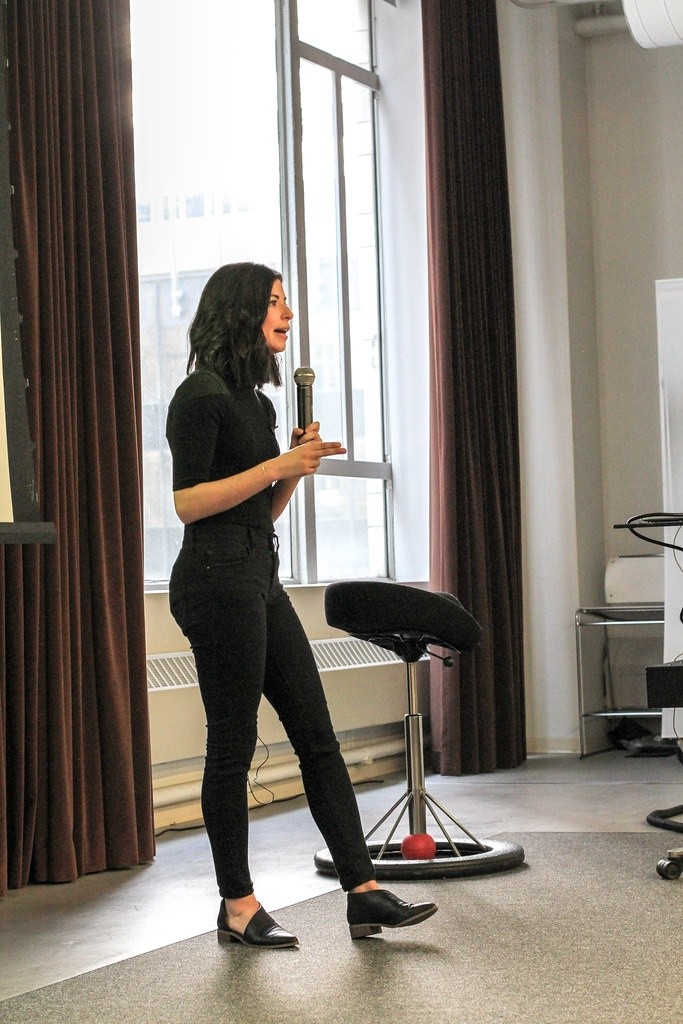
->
[347,888,438,939]
[217,899,298,948]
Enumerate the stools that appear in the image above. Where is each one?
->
[313,579,525,878]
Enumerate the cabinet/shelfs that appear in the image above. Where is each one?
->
[575,606,664,757]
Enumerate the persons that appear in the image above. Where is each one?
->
[166,261,438,951]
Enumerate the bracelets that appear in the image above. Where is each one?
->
[261,463,268,488]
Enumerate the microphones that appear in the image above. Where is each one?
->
[294,366,316,446]
